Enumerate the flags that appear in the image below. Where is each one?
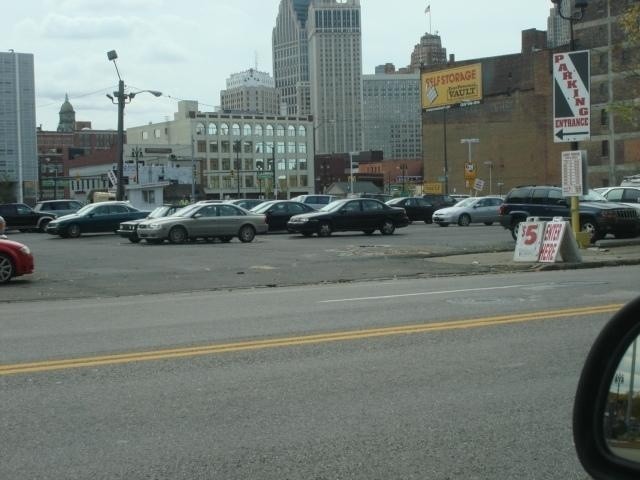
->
[424,4,431,14]
